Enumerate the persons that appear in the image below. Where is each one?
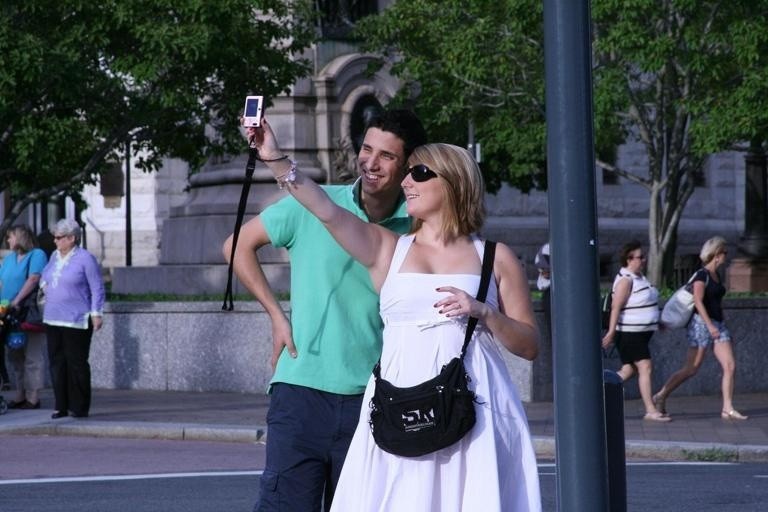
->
[220,107,430,511]
[234,111,544,512]
[652,235,751,420]
[0,223,50,411]
[533,238,552,342]
[38,217,106,420]
[597,239,672,423]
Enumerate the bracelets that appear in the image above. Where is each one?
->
[270,160,299,191]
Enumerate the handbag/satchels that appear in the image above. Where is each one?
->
[601,291,613,332]
[659,283,698,330]
[6,331,28,350]
[17,281,48,333]
[368,356,488,460]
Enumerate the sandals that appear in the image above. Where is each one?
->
[720,408,749,421]
[651,392,670,418]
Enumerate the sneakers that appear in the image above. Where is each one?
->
[51,408,69,418]
[20,399,41,410]
[6,398,27,409]
[643,410,673,422]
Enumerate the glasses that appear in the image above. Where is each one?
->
[53,234,66,241]
[400,164,438,183]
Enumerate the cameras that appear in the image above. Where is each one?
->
[242,95,263,126]
